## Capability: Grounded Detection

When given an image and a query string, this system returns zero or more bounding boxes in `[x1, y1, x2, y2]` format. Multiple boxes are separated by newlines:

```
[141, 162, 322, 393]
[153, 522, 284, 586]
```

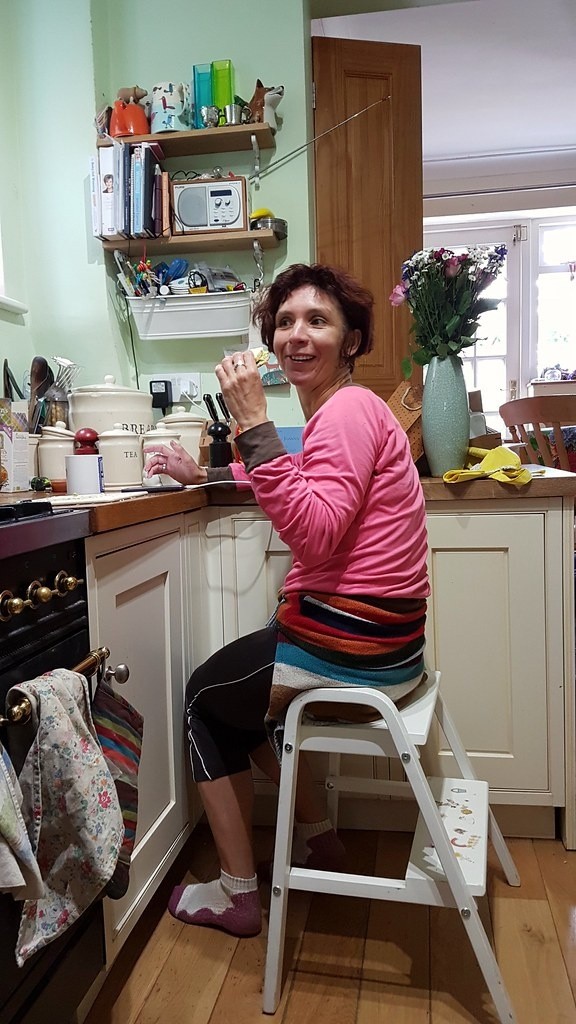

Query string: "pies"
[249, 347, 270, 367]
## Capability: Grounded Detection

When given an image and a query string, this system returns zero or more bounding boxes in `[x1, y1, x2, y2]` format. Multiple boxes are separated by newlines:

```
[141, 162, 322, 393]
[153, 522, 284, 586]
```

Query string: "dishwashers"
[0, 571, 130, 1011]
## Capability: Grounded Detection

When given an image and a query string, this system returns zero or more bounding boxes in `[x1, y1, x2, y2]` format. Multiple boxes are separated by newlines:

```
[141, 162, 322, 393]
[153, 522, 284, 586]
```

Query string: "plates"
[18, 491, 149, 505]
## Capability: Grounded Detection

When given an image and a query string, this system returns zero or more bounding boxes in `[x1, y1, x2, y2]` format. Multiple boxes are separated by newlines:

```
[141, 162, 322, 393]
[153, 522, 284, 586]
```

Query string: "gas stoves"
[0, 501, 93, 560]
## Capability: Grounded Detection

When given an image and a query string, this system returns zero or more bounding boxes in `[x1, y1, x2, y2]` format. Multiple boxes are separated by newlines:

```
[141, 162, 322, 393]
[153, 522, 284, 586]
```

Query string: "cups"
[27, 432, 42, 491]
[97, 405, 206, 489]
[108, 100, 149, 138]
[65, 454, 106, 494]
[37, 421, 77, 481]
[42, 400, 70, 429]
[192, 59, 252, 130]
[151, 80, 192, 134]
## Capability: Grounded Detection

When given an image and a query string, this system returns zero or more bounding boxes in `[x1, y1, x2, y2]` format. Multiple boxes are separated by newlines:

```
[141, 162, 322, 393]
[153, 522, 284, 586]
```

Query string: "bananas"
[249, 208, 275, 219]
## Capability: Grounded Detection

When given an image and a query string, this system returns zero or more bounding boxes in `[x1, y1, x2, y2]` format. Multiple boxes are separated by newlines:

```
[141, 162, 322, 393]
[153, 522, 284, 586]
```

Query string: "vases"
[423, 357, 470, 477]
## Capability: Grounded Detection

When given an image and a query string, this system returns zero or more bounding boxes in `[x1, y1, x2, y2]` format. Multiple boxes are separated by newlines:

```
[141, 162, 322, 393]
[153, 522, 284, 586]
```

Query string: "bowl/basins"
[69, 375, 155, 431]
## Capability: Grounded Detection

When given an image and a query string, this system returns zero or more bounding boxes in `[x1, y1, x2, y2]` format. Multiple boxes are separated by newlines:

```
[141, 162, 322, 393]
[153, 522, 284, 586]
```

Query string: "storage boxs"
[1, 431, 30, 493]
[130, 291, 252, 343]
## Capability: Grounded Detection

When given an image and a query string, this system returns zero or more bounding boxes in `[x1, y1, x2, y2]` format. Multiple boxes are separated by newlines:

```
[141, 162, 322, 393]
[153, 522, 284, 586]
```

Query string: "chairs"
[500, 392, 576, 471]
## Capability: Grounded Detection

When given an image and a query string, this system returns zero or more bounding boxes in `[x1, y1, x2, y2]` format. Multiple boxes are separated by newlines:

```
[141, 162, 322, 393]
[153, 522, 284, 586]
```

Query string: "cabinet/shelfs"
[85, 508, 213, 972]
[218, 499, 570, 842]
[97, 121, 279, 252]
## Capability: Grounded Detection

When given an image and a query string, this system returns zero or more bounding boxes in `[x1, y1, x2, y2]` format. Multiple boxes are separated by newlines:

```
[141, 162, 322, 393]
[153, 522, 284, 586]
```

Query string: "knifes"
[202, 392, 232, 431]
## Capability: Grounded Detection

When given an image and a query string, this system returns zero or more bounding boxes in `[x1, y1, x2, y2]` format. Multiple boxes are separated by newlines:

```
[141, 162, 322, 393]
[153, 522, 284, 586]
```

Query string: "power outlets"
[172, 372, 202, 404]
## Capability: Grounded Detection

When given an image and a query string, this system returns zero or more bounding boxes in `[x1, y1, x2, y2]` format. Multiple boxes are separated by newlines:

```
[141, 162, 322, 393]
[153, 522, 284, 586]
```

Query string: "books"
[92, 143, 171, 241]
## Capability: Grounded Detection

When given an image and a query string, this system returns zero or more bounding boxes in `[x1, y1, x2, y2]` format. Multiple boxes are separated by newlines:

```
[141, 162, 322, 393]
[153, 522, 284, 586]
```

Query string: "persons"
[136, 265, 432, 937]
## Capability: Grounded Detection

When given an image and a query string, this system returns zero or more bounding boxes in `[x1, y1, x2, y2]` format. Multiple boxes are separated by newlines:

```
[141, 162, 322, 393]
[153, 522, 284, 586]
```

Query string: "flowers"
[389, 245, 507, 379]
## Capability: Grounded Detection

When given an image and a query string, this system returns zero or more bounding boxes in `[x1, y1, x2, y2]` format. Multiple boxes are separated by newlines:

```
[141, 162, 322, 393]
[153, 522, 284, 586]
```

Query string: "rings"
[162, 463, 166, 473]
[233, 362, 247, 372]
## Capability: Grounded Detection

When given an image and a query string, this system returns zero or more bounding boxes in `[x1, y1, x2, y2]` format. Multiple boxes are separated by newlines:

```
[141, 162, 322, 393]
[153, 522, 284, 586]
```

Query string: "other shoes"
[266, 828, 347, 882]
[165, 884, 261, 937]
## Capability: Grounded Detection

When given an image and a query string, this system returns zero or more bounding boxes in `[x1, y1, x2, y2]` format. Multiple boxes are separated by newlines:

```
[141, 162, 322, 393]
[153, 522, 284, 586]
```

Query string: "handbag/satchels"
[386, 380, 426, 462]
